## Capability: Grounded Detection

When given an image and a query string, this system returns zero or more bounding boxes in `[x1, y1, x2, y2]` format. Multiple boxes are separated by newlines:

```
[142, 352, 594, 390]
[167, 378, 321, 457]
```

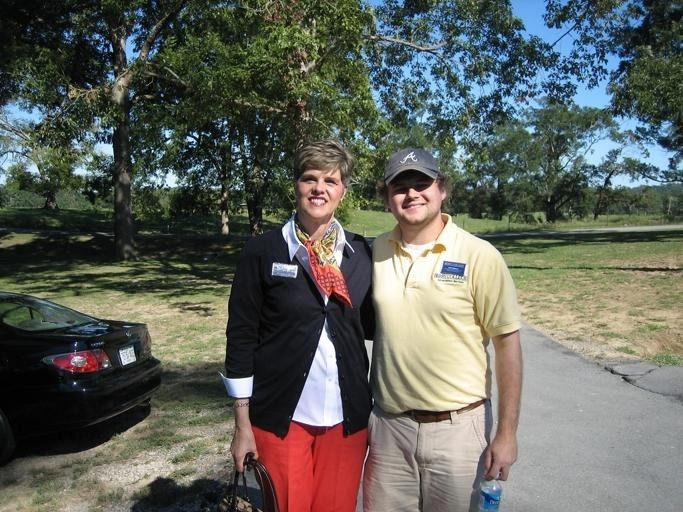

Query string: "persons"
[363, 148, 523, 512]
[218, 140, 375, 512]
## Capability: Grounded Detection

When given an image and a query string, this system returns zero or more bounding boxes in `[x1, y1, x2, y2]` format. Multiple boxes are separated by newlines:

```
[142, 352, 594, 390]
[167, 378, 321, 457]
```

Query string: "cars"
[0, 287, 166, 468]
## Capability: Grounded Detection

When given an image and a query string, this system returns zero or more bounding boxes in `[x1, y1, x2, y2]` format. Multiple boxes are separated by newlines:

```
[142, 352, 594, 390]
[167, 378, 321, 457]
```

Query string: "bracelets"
[231, 401, 249, 409]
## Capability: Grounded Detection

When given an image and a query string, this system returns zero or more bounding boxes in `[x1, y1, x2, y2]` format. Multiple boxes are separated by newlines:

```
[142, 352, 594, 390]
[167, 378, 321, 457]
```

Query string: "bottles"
[476, 476, 502, 512]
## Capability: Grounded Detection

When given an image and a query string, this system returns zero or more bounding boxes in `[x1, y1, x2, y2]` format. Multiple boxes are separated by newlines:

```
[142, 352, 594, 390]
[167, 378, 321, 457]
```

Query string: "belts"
[408, 400, 489, 421]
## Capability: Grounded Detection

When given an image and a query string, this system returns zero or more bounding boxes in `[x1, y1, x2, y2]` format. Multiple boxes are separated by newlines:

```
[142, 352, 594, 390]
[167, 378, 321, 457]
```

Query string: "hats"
[381, 148, 437, 184]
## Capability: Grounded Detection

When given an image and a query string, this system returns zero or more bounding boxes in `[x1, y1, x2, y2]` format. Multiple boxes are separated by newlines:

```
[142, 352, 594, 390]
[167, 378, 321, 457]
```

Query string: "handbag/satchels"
[219, 493, 261, 512]
